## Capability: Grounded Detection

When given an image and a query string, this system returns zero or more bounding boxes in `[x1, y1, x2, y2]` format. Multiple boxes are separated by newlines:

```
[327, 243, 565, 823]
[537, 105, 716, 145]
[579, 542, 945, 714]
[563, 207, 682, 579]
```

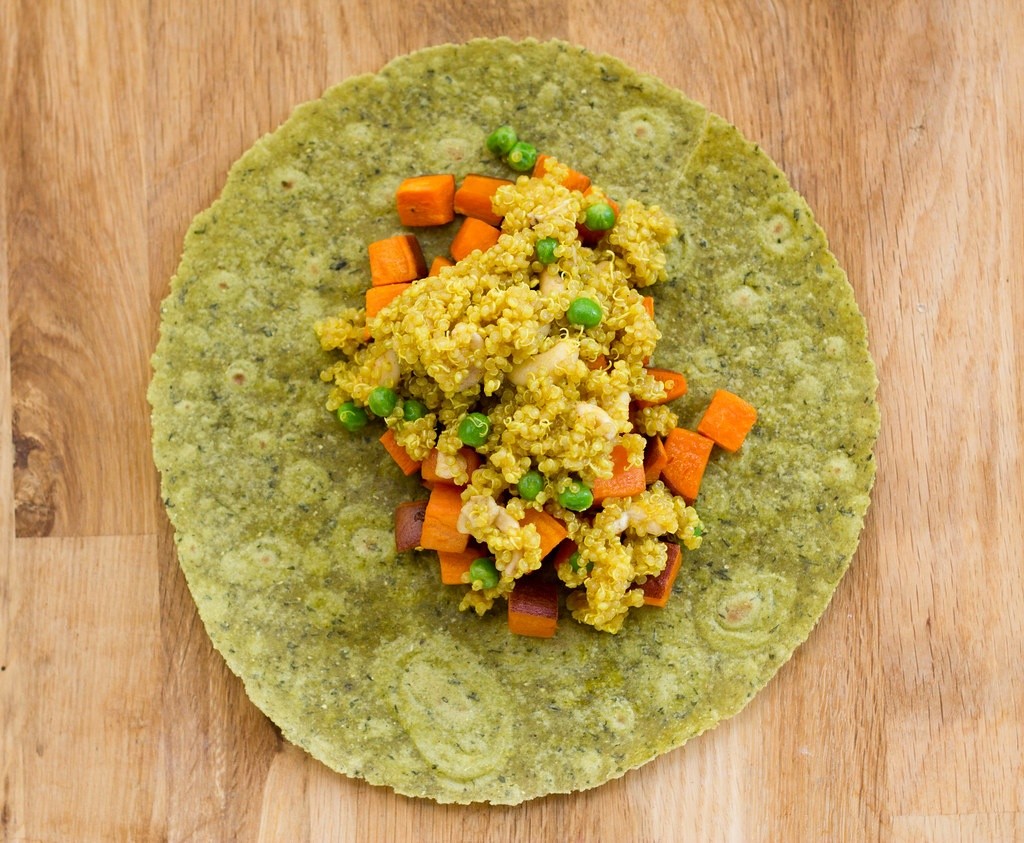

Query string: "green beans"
[336, 126, 615, 590]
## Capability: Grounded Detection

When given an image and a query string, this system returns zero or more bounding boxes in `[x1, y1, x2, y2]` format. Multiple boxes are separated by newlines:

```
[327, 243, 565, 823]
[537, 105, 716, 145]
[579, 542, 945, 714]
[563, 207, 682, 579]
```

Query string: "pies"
[146, 33, 883, 809]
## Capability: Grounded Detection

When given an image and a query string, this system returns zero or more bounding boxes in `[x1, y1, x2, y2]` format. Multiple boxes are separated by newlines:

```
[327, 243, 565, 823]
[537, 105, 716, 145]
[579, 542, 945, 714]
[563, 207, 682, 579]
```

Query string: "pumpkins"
[365, 157, 757, 640]
